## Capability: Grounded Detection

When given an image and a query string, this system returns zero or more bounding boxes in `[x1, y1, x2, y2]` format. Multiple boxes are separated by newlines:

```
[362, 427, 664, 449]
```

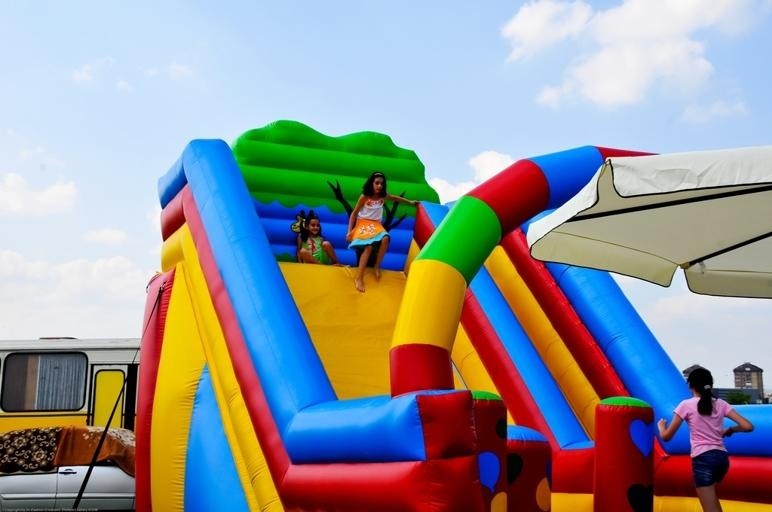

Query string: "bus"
[0, 334, 140, 435]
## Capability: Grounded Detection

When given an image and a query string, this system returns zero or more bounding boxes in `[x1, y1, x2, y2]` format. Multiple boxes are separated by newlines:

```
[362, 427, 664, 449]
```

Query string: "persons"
[346, 172, 421, 292]
[657, 369, 753, 512]
[297, 216, 343, 266]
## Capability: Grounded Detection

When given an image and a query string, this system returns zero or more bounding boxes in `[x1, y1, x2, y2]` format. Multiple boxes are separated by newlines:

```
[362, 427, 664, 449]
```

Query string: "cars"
[0, 426, 138, 512]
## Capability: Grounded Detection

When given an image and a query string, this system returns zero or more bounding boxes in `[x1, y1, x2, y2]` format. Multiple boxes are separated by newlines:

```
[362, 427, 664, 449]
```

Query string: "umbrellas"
[526, 146, 772, 299]
